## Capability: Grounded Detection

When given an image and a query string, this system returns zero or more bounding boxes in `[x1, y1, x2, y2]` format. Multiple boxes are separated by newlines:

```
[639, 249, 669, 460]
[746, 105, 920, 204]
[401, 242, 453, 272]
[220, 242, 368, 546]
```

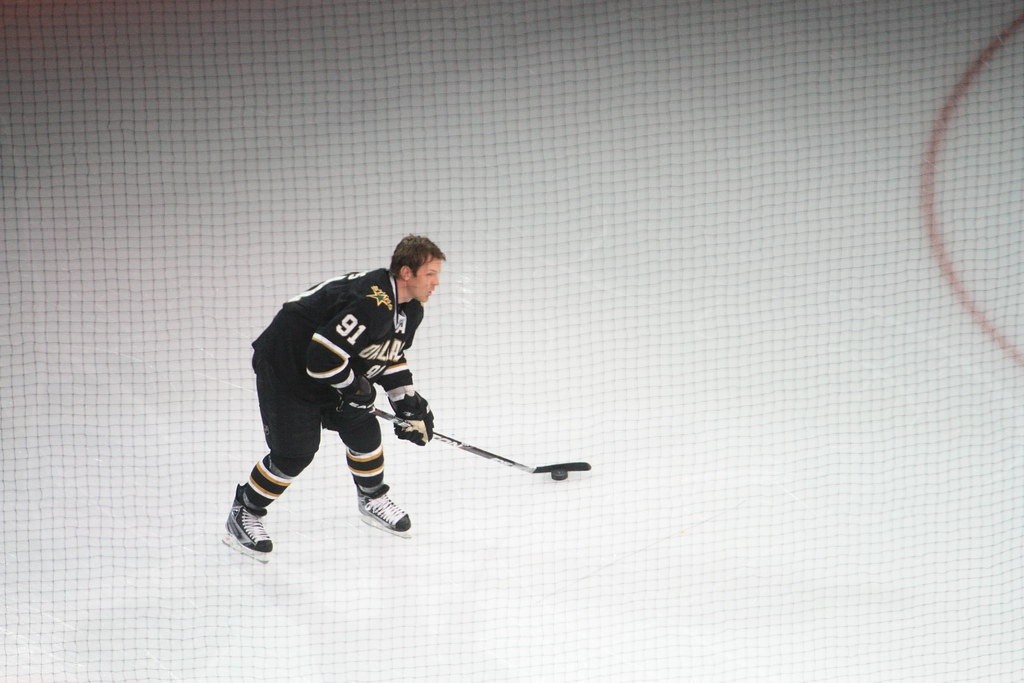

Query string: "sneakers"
[357, 486, 411, 538]
[222, 483, 273, 562]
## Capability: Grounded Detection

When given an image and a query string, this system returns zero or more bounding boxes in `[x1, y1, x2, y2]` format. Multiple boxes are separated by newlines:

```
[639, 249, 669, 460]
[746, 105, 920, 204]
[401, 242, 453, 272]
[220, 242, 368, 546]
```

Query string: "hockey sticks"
[370, 405, 593, 476]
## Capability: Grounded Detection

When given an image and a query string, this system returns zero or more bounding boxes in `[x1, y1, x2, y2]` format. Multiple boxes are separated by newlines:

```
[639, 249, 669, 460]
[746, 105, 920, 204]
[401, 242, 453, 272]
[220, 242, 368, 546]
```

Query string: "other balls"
[550, 468, 569, 482]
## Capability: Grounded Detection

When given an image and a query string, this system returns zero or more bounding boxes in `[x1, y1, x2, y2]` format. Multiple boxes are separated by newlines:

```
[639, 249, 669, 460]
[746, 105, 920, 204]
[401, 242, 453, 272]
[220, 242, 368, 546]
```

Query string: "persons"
[222, 235, 446, 562]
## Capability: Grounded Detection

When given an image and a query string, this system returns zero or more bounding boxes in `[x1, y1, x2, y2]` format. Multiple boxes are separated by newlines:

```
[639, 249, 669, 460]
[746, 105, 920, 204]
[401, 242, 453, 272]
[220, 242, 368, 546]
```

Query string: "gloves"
[388, 391, 434, 446]
[327, 372, 376, 413]
